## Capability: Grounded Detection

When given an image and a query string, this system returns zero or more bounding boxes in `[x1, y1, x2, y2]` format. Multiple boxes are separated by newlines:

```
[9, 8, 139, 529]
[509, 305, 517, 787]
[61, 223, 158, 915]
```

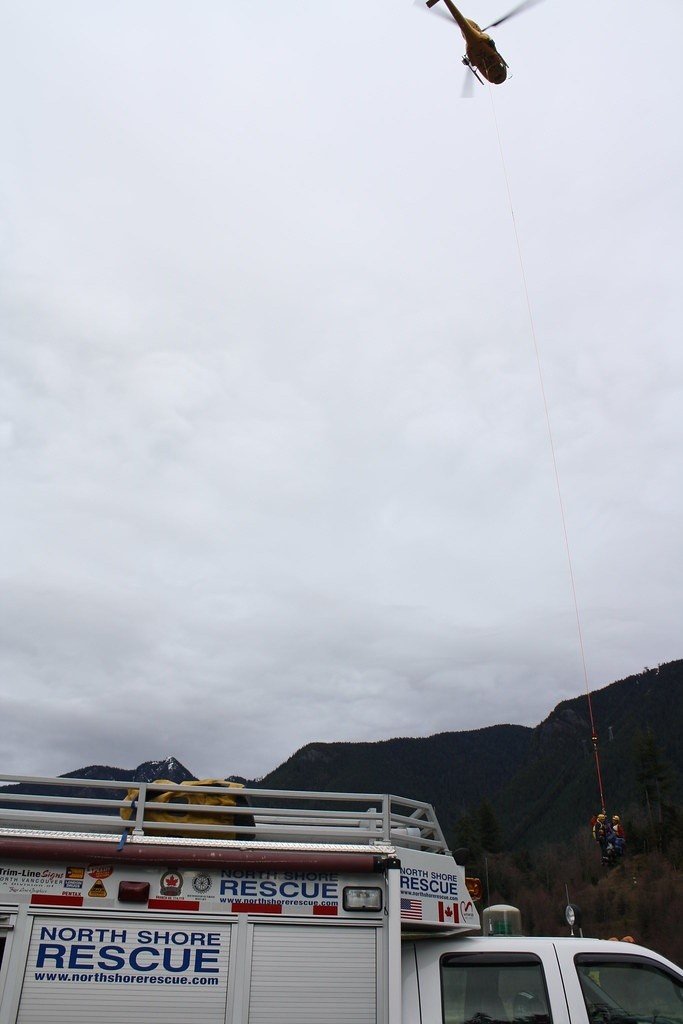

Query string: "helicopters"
[425, 0, 540, 88]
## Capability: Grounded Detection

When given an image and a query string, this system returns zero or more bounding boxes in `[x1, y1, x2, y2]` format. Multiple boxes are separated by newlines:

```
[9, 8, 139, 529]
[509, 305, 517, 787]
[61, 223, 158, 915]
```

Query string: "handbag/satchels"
[119, 779, 258, 842]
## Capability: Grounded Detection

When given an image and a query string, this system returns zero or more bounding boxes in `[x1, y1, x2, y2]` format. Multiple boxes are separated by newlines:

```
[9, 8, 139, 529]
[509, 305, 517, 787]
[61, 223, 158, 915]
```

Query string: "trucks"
[0, 771, 683, 1023]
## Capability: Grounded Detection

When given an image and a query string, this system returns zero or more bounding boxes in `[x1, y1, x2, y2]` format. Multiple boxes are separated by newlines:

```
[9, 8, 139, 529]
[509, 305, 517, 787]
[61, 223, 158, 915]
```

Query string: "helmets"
[612, 816, 619, 820]
[609, 937, 618, 941]
[598, 814, 605, 819]
[622, 936, 635, 943]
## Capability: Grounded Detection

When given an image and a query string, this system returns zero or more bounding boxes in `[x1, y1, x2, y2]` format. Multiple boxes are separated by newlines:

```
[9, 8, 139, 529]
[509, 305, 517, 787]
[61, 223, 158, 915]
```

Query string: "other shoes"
[600, 858, 604, 864]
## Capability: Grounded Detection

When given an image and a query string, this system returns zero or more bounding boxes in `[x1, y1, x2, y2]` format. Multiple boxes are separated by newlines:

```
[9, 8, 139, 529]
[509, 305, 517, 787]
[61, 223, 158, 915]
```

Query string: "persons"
[592, 811, 625, 867]
